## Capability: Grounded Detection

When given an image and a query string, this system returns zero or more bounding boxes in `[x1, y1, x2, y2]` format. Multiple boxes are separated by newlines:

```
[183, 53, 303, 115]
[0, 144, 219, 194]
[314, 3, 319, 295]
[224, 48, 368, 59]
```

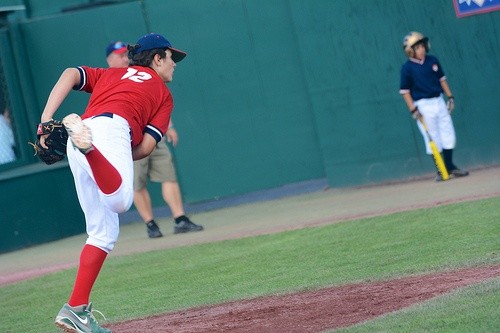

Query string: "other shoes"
[437, 170, 441, 181]
[451, 167, 469, 177]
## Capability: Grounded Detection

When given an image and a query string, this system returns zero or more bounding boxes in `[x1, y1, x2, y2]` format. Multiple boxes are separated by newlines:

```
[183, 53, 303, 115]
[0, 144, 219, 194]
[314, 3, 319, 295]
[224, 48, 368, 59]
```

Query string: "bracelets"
[447, 96, 454, 99]
[168, 125, 176, 129]
[408, 105, 418, 114]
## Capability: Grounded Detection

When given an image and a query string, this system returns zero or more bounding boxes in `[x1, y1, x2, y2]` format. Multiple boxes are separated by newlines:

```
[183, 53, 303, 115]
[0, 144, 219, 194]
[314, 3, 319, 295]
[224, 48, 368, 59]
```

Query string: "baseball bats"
[419, 115, 449, 181]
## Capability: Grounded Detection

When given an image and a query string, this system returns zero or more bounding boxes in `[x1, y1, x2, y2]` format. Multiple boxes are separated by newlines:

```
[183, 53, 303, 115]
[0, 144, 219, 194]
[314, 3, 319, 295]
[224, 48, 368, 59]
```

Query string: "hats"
[106, 41, 129, 57]
[128, 32, 187, 64]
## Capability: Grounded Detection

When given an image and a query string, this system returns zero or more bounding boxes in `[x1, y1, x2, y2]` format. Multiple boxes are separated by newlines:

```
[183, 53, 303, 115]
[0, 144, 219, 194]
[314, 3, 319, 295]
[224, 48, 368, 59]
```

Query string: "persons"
[33, 33, 187, 333]
[399, 30, 469, 182]
[104, 41, 203, 239]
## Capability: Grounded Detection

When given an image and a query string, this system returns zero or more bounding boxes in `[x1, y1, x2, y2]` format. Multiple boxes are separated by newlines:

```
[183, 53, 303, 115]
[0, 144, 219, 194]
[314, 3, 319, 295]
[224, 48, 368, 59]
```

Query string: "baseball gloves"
[29, 120, 69, 164]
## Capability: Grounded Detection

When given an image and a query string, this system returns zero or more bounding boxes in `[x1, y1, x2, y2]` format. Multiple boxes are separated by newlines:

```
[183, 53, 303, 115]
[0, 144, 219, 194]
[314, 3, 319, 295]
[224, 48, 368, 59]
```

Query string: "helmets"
[402, 31, 431, 58]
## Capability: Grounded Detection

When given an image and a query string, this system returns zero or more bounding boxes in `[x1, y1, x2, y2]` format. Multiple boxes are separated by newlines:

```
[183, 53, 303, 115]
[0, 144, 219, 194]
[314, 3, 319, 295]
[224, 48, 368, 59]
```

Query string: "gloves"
[412, 108, 423, 121]
[447, 97, 455, 114]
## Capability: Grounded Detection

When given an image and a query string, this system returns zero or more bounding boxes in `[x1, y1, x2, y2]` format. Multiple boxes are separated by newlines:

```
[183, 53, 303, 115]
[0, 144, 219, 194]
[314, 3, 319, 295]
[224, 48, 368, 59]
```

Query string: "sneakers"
[147, 225, 163, 238]
[53, 303, 113, 333]
[62, 113, 94, 152]
[174, 220, 204, 234]
